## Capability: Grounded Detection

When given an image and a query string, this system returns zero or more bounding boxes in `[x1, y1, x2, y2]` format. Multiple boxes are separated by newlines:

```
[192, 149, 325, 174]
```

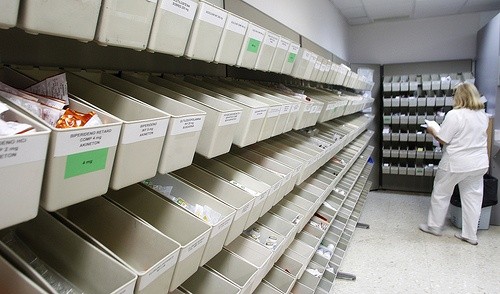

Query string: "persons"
[418, 81, 490, 245]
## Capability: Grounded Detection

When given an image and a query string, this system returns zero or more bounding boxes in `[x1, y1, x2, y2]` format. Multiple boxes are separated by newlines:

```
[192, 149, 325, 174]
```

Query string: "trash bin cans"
[449, 173, 499, 230]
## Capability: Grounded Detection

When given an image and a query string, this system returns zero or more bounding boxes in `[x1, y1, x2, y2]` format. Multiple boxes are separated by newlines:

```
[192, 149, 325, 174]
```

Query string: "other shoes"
[454, 231, 479, 245]
[419, 224, 441, 236]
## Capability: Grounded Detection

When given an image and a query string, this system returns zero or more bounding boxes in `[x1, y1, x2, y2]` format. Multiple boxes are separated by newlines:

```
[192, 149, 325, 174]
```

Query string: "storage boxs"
[0, 0, 376, 294]
[381, 72, 475, 178]
